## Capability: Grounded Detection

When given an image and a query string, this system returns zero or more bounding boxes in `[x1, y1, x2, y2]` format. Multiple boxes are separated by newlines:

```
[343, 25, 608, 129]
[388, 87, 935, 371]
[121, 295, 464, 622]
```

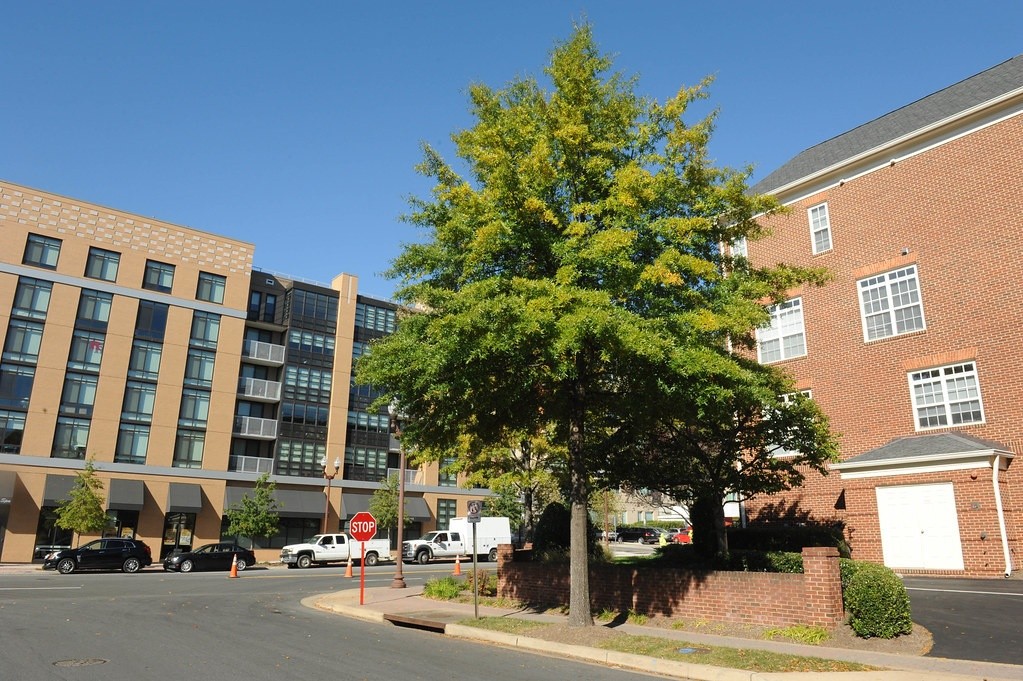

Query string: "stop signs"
[350, 512, 376, 542]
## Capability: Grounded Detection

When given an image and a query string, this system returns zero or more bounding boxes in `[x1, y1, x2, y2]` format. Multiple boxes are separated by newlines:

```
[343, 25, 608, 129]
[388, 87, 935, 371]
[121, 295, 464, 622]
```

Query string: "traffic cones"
[344, 556, 354, 579]
[451, 554, 464, 575]
[227, 554, 241, 578]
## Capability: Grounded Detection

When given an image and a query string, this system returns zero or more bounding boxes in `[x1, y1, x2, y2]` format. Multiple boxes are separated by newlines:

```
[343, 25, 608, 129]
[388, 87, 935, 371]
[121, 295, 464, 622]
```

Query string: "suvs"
[42, 537, 152, 574]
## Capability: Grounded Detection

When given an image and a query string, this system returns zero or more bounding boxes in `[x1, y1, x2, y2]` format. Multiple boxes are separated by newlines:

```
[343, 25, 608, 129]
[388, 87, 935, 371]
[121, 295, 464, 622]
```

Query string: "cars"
[162, 539, 255, 573]
[594, 529, 618, 540]
[616, 527, 692, 544]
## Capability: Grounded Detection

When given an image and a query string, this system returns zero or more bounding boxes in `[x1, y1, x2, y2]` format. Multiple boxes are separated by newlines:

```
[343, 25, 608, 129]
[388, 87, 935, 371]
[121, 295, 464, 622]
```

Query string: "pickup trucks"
[279, 534, 390, 569]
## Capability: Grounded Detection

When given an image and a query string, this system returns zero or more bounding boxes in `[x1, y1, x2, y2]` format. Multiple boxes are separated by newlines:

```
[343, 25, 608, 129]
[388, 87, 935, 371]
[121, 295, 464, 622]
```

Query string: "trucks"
[401, 516, 512, 565]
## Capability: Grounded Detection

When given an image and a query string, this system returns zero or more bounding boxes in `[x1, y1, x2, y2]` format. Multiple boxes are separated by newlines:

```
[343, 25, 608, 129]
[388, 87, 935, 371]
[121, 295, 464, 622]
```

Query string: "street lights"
[388, 397, 419, 588]
[319, 456, 341, 567]
